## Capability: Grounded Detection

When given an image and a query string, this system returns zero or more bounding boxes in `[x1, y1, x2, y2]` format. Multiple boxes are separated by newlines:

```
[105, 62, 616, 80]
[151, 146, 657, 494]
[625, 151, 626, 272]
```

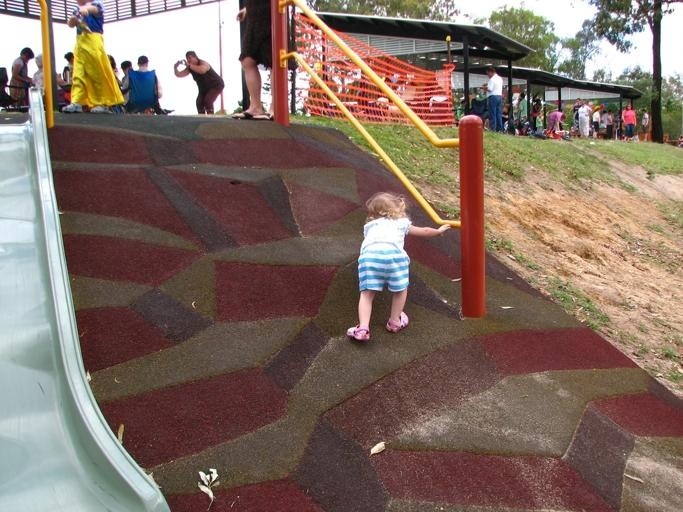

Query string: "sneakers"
[91, 105, 108, 113]
[62, 104, 82, 112]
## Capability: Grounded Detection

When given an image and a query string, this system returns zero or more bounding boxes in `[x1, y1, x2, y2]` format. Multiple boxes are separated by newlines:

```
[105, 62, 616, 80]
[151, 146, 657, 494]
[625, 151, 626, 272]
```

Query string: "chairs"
[0, 67, 25, 112]
[122, 69, 158, 114]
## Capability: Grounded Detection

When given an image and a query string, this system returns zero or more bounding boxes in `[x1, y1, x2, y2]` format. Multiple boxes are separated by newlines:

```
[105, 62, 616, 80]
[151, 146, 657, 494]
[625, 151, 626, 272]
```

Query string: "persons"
[62, 0, 124, 113]
[347, 192, 451, 341]
[10, 47, 162, 106]
[174, 51, 225, 115]
[458, 67, 652, 143]
[232, 0, 299, 121]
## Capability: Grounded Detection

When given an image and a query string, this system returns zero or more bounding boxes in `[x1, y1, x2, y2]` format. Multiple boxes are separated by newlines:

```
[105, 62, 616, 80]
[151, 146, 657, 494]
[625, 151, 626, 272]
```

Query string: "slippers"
[232, 111, 269, 119]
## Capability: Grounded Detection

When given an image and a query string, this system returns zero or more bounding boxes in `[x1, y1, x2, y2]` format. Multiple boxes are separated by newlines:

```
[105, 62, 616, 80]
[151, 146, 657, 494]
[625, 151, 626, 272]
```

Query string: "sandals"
[346, 325, 370, 341]
[387, 312, 409, 332]
